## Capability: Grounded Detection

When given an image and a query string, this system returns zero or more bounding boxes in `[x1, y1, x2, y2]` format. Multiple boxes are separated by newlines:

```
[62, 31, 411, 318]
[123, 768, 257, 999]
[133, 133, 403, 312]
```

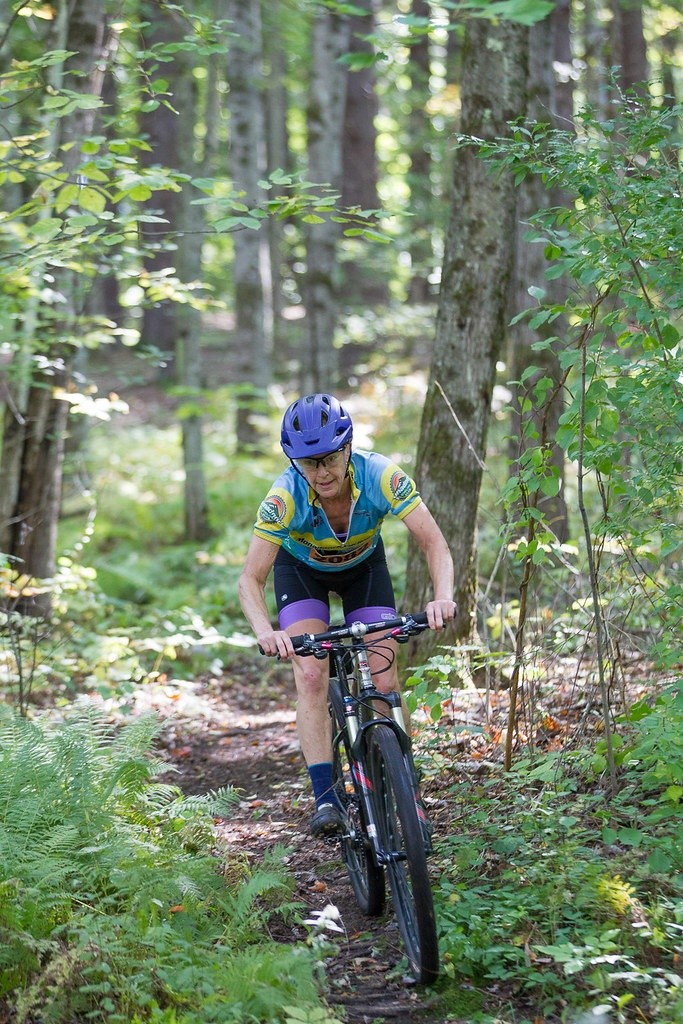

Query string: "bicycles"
[260, 606, 458, 988]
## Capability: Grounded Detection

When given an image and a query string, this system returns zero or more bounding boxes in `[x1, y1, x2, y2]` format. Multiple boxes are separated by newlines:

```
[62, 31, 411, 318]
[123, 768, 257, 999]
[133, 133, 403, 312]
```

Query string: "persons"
[237, 393, 456, 857]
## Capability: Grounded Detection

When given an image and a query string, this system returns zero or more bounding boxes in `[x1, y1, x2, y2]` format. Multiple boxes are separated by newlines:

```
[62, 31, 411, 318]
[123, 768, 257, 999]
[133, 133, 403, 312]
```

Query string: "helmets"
[280, 393, 353, 459]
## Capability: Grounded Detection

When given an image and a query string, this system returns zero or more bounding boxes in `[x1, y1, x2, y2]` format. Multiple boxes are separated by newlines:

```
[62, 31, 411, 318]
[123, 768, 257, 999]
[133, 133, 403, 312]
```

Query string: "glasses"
[297, 448, 344, 470]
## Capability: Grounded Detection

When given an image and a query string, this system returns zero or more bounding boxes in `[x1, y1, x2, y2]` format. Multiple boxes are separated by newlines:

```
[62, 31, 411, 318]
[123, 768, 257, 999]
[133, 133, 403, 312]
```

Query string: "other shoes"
[311, 807, 349, 842]
[420, 797, 433, 836]
